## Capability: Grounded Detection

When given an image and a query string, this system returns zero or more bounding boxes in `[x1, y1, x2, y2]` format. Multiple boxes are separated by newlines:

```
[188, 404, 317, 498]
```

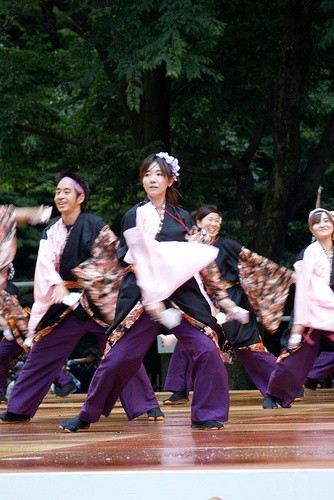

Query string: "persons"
[0, 203, 101, 403]
[58, 152, 230, 431]
[162, 206, 297, 405]
[262, 185, 334, 409]
[0, 172, 165, 425]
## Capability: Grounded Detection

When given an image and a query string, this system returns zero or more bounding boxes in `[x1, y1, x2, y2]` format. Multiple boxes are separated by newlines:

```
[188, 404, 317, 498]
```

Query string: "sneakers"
[163, 391, 190, 405]
[303, 378, 318, 393]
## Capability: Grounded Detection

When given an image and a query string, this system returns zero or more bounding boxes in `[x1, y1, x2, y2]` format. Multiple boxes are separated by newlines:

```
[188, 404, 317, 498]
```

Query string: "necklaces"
[322, 246, 333, 258]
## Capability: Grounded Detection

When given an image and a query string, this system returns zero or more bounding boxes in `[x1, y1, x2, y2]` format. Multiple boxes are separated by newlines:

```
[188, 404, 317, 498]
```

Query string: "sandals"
[293, 387, 306, 401]
[262, 397, 278, 410]
[190, 420, 225, 430]
[0, 412, 32, 422]
[55, 380, 77, 399]
[0, 396, 8, 404]
[58, 417, 90, 433]
[147, 407, 166, 421]
